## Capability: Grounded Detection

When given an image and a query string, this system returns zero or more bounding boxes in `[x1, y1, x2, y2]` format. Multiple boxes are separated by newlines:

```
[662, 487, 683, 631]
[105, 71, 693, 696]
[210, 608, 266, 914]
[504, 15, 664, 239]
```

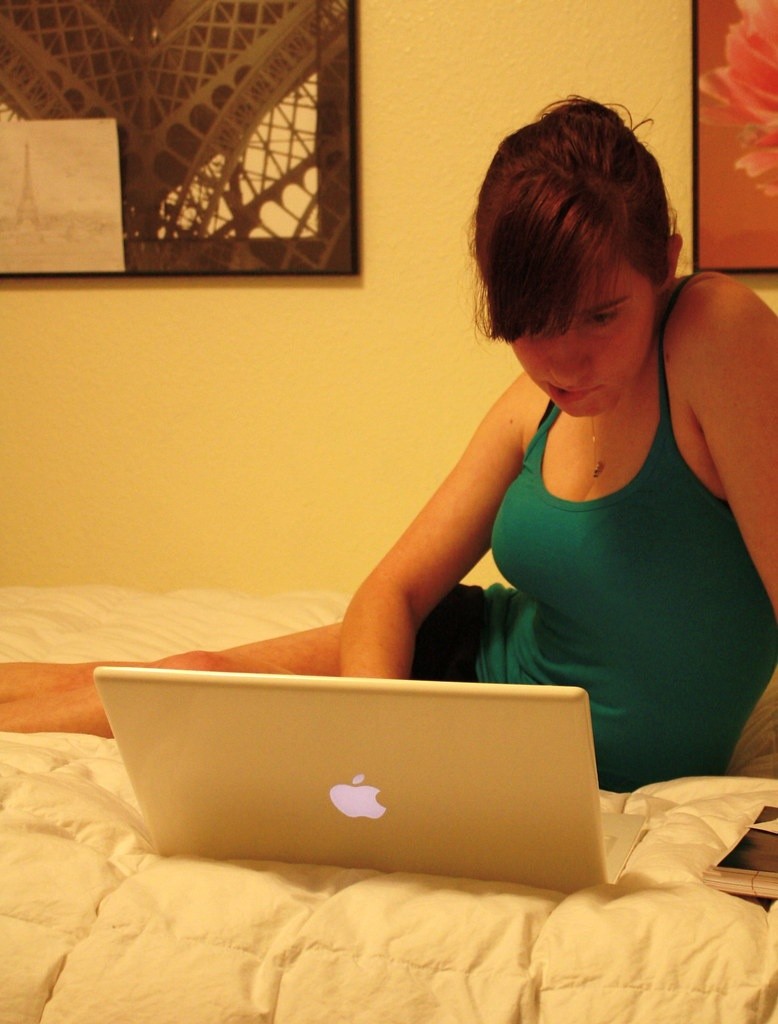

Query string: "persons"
[0, 95, 778, 793]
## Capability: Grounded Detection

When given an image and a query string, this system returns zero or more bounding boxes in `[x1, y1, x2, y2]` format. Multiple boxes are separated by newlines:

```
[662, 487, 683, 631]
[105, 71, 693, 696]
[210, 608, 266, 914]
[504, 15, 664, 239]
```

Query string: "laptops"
[94, 665, 648, 895]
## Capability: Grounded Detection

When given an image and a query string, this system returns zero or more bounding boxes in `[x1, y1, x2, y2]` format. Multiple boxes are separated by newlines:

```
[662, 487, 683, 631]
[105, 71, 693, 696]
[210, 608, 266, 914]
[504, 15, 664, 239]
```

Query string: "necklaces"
[592, 404, 653, 477]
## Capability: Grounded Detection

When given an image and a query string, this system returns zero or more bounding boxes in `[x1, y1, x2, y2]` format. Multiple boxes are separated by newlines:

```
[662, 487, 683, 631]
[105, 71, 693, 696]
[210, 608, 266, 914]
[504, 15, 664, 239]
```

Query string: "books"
[701, 805, 778, 900]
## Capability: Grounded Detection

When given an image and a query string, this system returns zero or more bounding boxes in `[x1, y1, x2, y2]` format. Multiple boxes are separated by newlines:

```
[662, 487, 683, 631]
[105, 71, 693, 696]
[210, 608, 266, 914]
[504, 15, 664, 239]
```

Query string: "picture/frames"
[692, 0, 778, 276]
[0, 0, 362, 278]
